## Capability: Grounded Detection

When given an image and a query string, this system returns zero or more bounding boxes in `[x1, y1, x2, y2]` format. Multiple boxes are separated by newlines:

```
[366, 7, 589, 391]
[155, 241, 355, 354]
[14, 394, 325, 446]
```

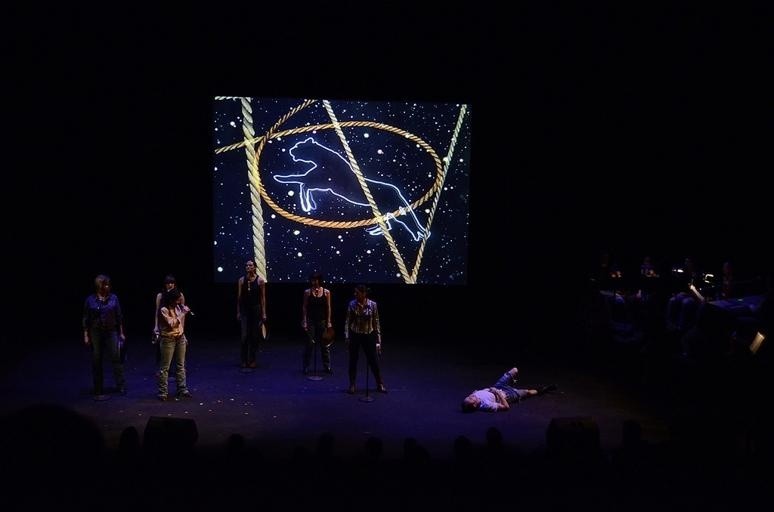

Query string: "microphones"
[179, 304, 195, 317]
[363, 302, 373, 313]
[312, 283, 319, 292]
[97, 296, 104, 308]
[245, 271, 251, 279]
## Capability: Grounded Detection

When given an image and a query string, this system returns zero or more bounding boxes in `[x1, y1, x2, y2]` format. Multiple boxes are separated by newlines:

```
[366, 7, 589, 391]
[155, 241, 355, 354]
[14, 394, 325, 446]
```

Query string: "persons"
[235, 260, 268, 368]
[154, 289, 192, 401]
[598, 256, 734, 313]
[462, 365, 557, 414]
[342, 284, 388, 394]
[80, 273, 129, 400]
[151, 276, 188, 376]
[299, 272, 334, 374]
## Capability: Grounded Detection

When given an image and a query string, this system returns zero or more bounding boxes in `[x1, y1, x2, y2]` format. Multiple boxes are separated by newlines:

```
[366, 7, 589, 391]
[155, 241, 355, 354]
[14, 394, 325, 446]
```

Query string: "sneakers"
[239, 359, 248, 368]
[156, 391, 169, 401]
[346, 382, 357, 394]
[248, 358, 257, 368]
[375, 384, 388, 394]
[177, 389, 193, 399]
